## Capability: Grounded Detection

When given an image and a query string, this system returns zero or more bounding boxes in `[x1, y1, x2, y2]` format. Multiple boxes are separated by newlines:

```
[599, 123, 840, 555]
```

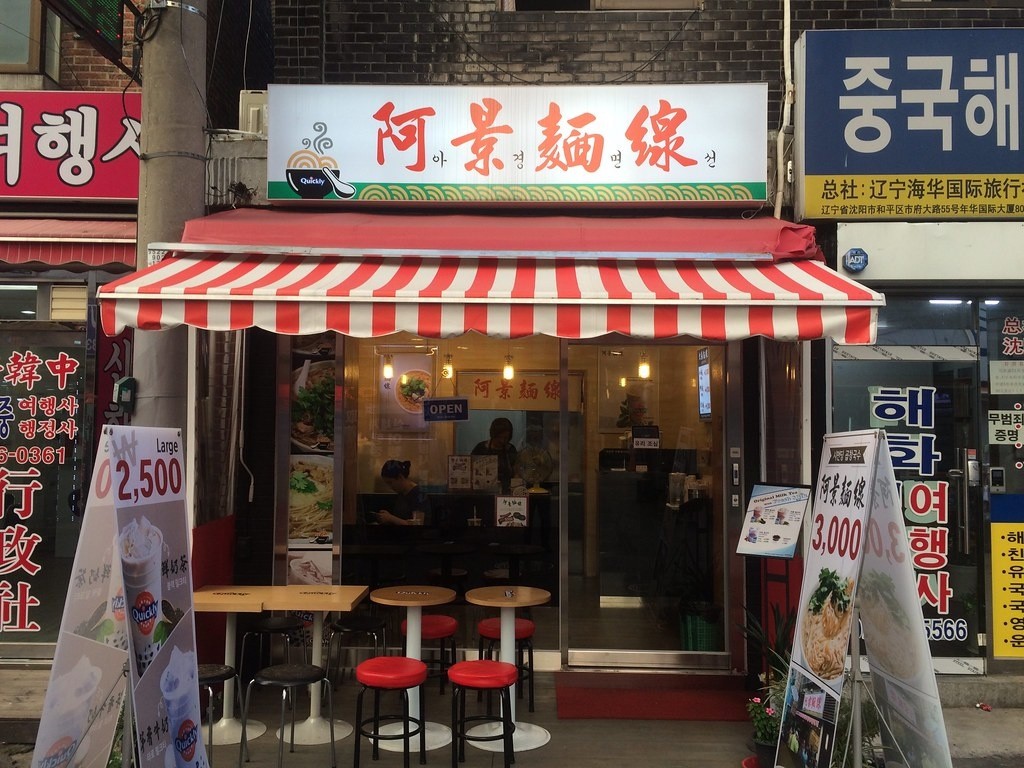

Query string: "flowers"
[746, 697, 786, 744]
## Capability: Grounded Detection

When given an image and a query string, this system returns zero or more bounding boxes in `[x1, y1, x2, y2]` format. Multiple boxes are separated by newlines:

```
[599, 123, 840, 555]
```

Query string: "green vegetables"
[402, 377, 425, 395]
[808, 567, 853, 610]
[290, 471, 333, 509]
[289, 373, 337, 439]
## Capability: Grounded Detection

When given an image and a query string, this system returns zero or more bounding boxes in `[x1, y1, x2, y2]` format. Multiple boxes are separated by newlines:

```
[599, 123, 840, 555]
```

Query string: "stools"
[321, 615, 389, 709]
[447, 660, 519, 768]
[235, 616, 310, 711]
[353, 657, 428, 768]
[399, 614, 459, 700]
[196, 664, 250, 768]
[476, 618, 536, 718]
[238, 663, 337, 768]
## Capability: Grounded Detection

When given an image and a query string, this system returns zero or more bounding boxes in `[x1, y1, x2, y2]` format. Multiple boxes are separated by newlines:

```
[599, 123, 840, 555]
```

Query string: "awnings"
[95, 244, 884, 345]
[0, 219, 137, 267]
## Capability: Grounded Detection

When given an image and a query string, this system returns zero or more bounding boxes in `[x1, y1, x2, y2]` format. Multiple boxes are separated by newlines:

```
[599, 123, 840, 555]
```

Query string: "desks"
[464, 586, 551, 753]
[341, 541, 548, 590]
[262, 586, 370, 745]
[192, 584, 287, 746]
[369, 585, 456, 752]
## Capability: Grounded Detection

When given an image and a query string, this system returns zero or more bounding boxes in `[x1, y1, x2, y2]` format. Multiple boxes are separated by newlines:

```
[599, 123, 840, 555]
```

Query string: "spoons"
[322, 167, 356, 199]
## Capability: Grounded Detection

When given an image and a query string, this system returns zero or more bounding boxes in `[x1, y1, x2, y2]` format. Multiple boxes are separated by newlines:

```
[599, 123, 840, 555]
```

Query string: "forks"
[285, 169, 340, 199]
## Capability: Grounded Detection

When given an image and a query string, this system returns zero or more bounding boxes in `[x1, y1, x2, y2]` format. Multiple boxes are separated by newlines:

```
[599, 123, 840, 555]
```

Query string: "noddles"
[289, 461, 335, 537]
[802, 577, 852, 679]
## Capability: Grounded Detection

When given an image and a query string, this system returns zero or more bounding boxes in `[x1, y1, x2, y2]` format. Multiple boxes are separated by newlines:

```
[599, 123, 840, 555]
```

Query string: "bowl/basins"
[288, 455, 333, 544]
[396, 369, 432, 414]
[290, 360, 334, 453]
[800, 579, 843, 687]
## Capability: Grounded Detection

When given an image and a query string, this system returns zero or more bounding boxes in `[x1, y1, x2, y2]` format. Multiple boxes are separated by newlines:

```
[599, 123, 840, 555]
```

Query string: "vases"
[748, 730, 779, 768]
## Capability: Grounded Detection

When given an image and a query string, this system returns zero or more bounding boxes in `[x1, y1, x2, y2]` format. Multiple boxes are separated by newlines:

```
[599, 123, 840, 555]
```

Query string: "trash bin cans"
[679, 597, 723, 651]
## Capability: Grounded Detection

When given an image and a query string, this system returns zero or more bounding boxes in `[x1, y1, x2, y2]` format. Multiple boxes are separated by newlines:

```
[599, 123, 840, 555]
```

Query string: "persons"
[470, 418, 518, 493]
[375, 460, 430, 580]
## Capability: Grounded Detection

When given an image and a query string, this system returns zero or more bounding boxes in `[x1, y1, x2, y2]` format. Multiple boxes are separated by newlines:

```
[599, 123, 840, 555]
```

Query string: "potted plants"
[734, 601, 799, 713]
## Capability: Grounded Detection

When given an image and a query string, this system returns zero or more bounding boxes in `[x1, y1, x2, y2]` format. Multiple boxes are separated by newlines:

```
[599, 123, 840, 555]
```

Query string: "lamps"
[502, 340, 515, 381]
[638, 338, 651, 379]
[382, 335, 395, 379]
[441, 339, 454, 379]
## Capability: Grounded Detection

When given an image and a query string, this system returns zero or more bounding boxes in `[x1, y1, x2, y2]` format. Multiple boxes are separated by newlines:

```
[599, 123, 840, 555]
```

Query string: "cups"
[669, 473, 709, 505]
[407, 520, 417, 525]
[104, 536, 128, 650]
[32, 665, 102, 768]
[777, 510, 788, 525]
[120, 524, 163, 677]
[467, 518, 482, 527]
[287, 560, 327, 625]
[161, 652, 203, 768]
[748, 528, 760, 543]
[754, 509, 764, 522]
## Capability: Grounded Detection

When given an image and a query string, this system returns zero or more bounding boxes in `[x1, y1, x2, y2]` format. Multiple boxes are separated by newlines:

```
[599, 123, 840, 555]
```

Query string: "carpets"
[554, 671, 770, 723]
